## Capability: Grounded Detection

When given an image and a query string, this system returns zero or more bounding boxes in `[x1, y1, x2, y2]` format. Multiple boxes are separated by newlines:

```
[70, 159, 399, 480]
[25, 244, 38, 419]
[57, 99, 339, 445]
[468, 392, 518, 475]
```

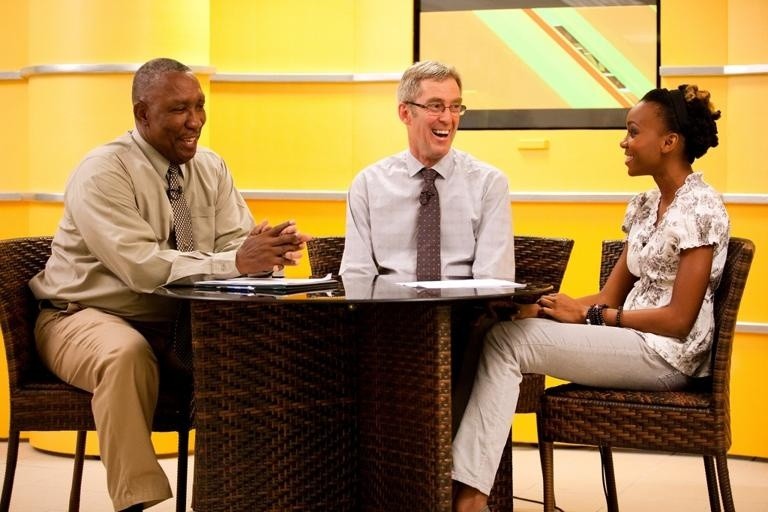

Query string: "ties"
[169, 164, 194, 367]
[417, 169, 441, 281]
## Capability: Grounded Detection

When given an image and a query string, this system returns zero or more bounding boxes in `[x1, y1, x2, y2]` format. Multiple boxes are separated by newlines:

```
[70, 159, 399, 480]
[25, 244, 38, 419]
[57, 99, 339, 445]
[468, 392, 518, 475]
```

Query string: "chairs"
[306, 236, 345, 298]
[481, 236, 582, 512]
[0, 237, 190, 512]
[539, 237, 755, 512]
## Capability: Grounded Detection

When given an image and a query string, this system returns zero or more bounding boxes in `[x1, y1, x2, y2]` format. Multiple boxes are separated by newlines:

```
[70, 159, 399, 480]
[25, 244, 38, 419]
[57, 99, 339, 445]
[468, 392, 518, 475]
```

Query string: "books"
[193, 275, 339, 295]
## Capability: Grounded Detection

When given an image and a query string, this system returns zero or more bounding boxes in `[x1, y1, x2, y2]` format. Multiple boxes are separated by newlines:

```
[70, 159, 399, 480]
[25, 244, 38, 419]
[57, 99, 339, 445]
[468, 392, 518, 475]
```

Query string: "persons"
[338, 56, 516, 284]
[451, 84, 731, 512]
[28, 59, 312, 511]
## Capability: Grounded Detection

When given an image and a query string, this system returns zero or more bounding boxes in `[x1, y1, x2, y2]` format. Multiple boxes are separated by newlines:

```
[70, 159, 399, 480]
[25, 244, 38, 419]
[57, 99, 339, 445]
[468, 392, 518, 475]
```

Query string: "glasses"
[407, 102, 466, 117]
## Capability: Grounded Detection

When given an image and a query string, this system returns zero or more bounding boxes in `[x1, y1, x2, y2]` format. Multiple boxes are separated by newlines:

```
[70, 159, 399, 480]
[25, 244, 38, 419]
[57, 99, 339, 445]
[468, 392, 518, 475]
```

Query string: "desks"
[154, 274, 554, 512]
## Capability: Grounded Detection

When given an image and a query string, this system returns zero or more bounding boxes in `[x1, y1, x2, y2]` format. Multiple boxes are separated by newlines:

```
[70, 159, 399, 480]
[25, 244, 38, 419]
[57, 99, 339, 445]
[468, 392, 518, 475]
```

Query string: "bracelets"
[615, 306, 624, 326]
[586, 303, 609, 326]
